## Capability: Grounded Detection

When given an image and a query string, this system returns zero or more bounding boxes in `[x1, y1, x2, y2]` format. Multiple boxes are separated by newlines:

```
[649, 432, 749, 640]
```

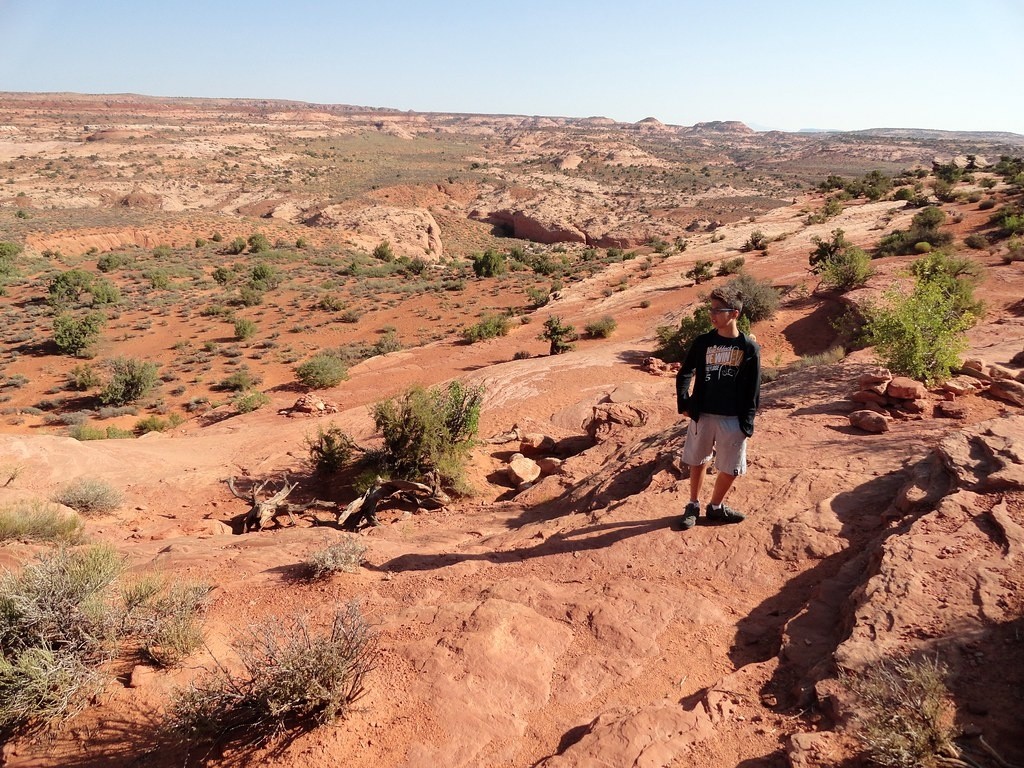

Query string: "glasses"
[707, 307, 735, 315]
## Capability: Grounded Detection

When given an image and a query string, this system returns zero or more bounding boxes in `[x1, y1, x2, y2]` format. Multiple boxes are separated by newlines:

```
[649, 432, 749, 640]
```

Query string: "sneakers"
[705, 503, 745, 523]
[681, 499, 700, 528]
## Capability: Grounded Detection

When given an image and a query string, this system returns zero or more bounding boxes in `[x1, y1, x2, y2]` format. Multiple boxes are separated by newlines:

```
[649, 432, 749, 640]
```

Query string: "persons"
[675, 285, 760, 528]
